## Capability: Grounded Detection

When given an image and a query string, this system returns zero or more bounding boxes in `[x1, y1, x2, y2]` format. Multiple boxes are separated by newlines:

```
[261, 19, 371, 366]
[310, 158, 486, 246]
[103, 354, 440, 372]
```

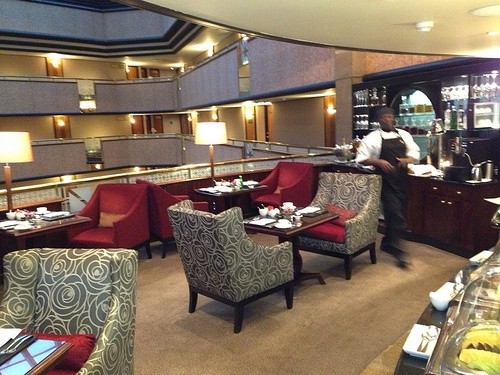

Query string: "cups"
[6, 207, 47, 221]
[259, 202, 301, 222]
[400, 104, 433, 113]
[215, 179, 240, 191]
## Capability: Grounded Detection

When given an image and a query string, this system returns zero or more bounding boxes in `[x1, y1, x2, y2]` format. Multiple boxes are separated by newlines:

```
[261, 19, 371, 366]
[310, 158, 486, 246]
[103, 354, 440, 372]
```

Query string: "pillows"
[21, 331, 95, 371]
[328, 205, 357, 227]
[98, 211, 126, 227]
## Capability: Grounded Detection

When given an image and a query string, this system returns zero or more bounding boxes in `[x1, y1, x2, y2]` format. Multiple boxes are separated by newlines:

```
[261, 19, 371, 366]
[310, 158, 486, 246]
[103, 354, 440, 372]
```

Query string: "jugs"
[471, 163, 483, 181]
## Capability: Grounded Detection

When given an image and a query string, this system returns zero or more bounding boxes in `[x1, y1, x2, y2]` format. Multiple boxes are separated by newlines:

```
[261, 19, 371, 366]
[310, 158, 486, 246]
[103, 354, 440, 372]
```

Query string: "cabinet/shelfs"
[351, 58, 500, 168]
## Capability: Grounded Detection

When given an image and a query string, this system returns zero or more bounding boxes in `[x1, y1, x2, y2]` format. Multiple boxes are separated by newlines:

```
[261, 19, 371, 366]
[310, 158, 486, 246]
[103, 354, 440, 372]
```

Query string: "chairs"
[0, 247, 138, 375]
[249, 161, 313, 208]
[296, 172, 383, 280]
[135, 179, 208, 258]
[168, 199, 295, 334]
[59, 183, 152, 260]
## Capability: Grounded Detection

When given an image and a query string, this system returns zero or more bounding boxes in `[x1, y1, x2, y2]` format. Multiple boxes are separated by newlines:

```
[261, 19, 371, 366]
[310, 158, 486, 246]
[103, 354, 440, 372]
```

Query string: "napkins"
[0, 334, 38, 366]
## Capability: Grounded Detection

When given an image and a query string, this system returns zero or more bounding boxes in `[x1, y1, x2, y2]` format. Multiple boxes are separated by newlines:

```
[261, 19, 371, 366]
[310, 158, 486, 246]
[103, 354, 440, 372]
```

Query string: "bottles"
[239, 176, 243, 188]
[444, 102, 467, 130]
[485, 160, 493, 180]
[354, 87, 386, 107]
[394, 118, 434, 135]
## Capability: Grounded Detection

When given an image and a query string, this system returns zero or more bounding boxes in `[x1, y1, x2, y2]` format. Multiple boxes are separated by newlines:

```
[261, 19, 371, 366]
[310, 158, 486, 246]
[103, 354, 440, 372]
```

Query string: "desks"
[0, 215, 92, 250]
[0, 339, 74, 375]
[194, 185, 268, 214]
[243, 211, 339, 285]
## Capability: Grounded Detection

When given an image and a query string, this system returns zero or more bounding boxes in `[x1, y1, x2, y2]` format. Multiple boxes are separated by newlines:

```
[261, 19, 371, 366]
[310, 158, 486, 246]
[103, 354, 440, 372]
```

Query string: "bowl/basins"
[335, 149, 353, 161]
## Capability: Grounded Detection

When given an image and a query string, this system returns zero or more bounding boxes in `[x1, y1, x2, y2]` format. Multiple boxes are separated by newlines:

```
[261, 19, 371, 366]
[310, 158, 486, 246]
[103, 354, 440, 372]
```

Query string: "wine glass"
[354, 114, 368, 129]
[441, 85, 469, 100]
[471, 73, 500, 98]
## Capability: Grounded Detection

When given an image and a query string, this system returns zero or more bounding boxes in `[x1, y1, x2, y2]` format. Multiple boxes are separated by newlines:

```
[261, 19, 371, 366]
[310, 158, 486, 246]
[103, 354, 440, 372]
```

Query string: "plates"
[402, 324, 441, 359]
[249, 206, 321, 229]
[198, 181, 258, 193]
[469, 251, 494, 265]
[0, 212, 70, 230]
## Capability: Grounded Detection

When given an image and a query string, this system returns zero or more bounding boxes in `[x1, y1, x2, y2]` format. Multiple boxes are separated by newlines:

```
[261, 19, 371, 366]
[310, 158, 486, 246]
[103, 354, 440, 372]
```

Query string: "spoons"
[0, 335, 33, 354]
[416, 326, 439, 353]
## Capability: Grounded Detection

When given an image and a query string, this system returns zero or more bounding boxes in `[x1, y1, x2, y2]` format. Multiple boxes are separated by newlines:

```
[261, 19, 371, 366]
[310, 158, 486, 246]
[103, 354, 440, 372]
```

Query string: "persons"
[355, 106, 421, 268]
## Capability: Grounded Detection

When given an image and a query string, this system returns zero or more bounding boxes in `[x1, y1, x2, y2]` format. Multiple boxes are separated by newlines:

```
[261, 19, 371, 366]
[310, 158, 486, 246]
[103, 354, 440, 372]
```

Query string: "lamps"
[0, 131, 33, 211]
[194, 122, 228, 186]
